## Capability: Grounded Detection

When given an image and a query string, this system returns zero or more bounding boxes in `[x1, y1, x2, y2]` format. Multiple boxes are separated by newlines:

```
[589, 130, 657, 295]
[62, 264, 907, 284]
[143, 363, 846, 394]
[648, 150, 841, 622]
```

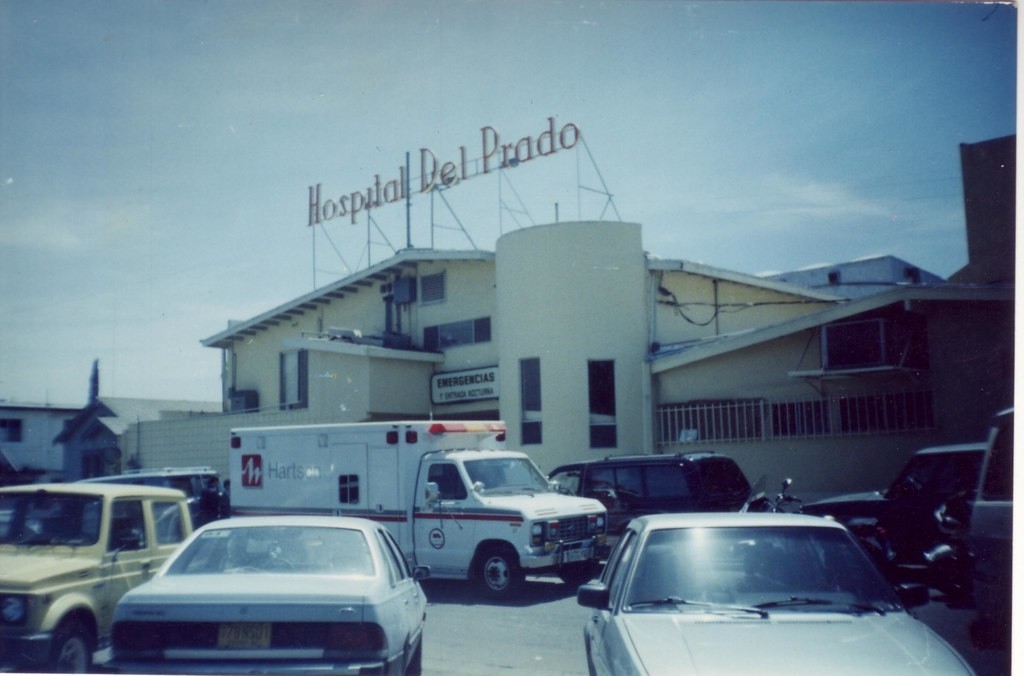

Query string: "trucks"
[226, 420, 608, 600]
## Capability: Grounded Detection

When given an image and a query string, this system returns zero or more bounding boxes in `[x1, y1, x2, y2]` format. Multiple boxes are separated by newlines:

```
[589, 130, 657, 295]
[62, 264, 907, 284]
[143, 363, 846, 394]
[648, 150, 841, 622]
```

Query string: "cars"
[1, 482, 428, 673]
[578, 407, 1011, 676]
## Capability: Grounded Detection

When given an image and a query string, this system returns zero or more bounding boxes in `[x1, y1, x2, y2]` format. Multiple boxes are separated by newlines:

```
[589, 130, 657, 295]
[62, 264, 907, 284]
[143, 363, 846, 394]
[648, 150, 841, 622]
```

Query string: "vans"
[549, 450, 752, 560]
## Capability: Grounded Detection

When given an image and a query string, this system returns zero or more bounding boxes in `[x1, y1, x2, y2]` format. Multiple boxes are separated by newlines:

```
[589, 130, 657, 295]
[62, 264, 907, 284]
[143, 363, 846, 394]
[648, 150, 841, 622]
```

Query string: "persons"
[199, 477, 222, 527]
[218, 480, 230, 519]
